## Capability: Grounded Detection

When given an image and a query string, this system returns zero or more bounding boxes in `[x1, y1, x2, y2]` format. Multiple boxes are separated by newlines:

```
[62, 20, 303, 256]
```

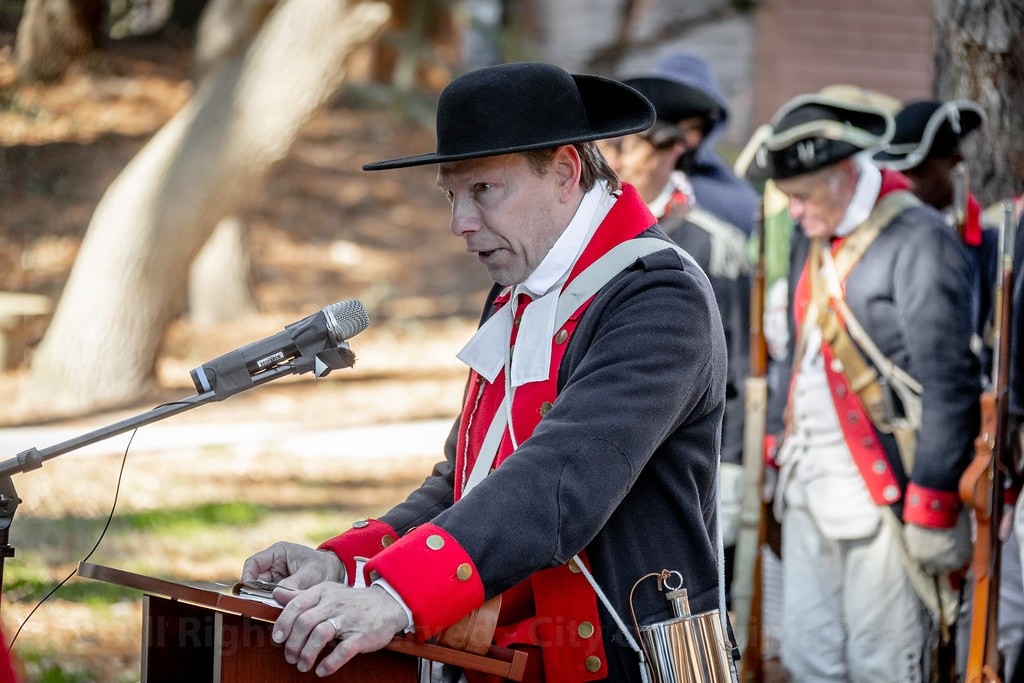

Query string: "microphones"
[188, 298, 370, 394]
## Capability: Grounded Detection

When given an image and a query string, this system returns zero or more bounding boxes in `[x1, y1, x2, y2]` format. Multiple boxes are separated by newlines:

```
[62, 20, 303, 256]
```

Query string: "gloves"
[902, 524, 975, 576]
[716, 463, 745, 551]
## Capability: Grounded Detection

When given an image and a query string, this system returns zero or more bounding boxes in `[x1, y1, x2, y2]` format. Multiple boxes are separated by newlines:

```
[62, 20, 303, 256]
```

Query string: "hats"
[731, 89, 896, 180]
[618, 74, 730, 153]
[871, 93, 991, 171]
[360, 61, 658, 171]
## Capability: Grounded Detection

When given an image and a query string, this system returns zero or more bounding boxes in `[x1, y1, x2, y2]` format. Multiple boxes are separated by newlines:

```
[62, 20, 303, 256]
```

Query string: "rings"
[324, 619, 343, 638]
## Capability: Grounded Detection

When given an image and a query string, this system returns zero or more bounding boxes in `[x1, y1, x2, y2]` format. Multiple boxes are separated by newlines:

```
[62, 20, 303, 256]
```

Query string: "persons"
[239, 62, 742, 683]
[585, 53, 1024, 683]
